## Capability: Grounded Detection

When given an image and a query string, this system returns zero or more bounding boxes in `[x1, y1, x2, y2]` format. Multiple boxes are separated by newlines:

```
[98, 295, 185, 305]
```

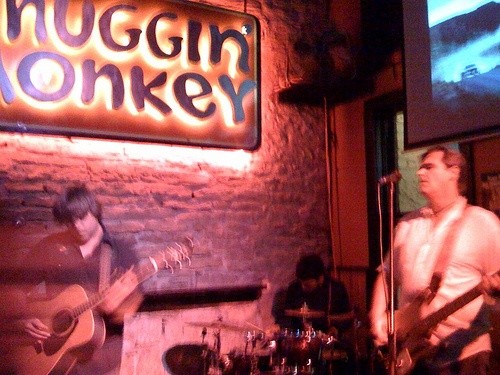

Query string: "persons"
[0, 178, 145, 375]
[282, 254, 357, 375]
[370, 144, 500, 375]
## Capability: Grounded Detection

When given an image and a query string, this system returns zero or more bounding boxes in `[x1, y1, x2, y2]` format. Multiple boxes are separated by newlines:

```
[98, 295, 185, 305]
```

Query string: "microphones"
[376, 169, 402, 187]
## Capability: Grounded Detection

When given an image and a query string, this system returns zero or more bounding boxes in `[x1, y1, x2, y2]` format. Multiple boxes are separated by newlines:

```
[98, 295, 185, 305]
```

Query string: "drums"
[223, 330, 352, 375]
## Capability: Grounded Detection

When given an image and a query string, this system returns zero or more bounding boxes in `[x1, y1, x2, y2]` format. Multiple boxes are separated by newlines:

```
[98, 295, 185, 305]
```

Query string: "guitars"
[8, 236, 194, 375]
[371, 271, 500, 375]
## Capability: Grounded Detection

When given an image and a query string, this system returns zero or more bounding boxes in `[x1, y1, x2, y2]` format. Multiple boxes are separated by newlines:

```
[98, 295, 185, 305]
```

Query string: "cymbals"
[163, 344, 215, 375]
[286, 307, 323, 318]
[188, 322, 244, 332]
